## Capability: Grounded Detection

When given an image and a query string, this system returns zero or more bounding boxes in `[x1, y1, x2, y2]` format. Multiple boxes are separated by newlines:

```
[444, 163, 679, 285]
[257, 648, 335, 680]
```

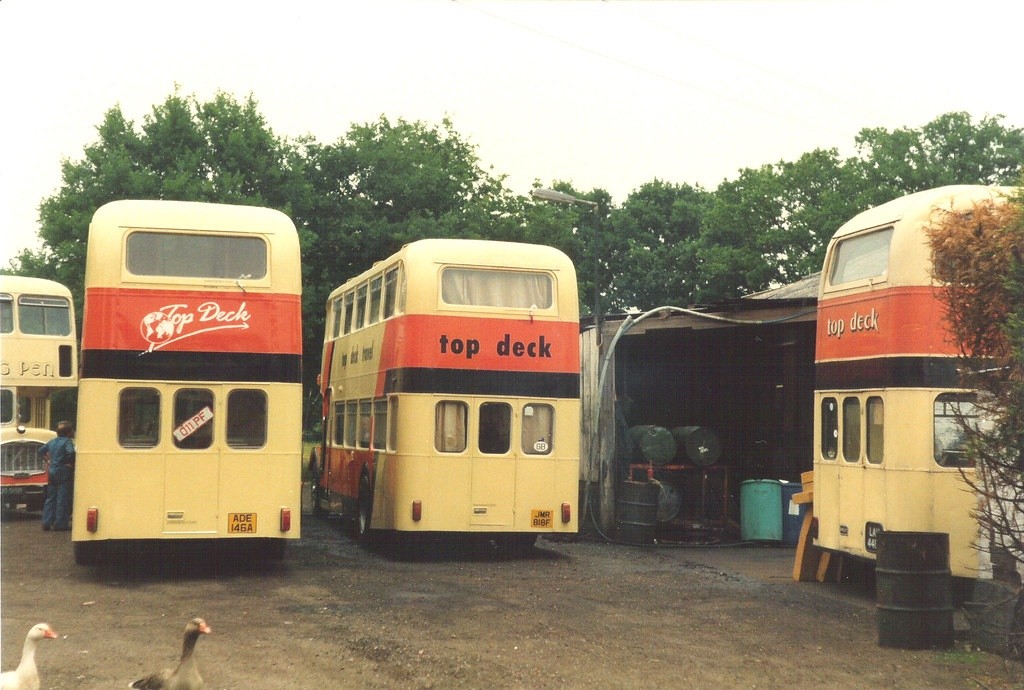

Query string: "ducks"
[1, 622, 58, 690]
[129, 617, 213, 690]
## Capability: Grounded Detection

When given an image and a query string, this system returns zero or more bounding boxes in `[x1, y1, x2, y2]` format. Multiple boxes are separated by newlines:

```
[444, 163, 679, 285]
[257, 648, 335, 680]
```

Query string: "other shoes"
[42, 524, 50, 530]
[54, 526, 72, 531]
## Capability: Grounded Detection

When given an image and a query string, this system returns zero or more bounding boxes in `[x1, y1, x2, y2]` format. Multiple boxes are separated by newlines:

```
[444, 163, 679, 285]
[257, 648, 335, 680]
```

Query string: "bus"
[70, 199, 303, 564]
[311, 238, 582, 548]
[0, 275, 78, 511]
[810, 184, 1024, 578]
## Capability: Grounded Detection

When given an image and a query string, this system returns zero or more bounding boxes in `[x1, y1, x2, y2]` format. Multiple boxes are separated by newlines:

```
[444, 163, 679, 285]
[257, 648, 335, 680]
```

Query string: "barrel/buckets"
[876, 529, 956, 651]
[619, 480, 660, 544]
[738, 478, 805, 545]
[626, 424, 724, 467]
[658, 481, 690, 522]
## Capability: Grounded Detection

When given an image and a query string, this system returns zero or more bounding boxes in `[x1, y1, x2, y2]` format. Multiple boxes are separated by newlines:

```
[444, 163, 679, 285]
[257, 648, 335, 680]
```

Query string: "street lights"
[533, 187, 602, 353]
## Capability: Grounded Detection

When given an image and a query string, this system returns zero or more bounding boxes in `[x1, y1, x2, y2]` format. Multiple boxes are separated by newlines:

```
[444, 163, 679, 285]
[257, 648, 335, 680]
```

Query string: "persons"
[36, 420, 75, 531]
